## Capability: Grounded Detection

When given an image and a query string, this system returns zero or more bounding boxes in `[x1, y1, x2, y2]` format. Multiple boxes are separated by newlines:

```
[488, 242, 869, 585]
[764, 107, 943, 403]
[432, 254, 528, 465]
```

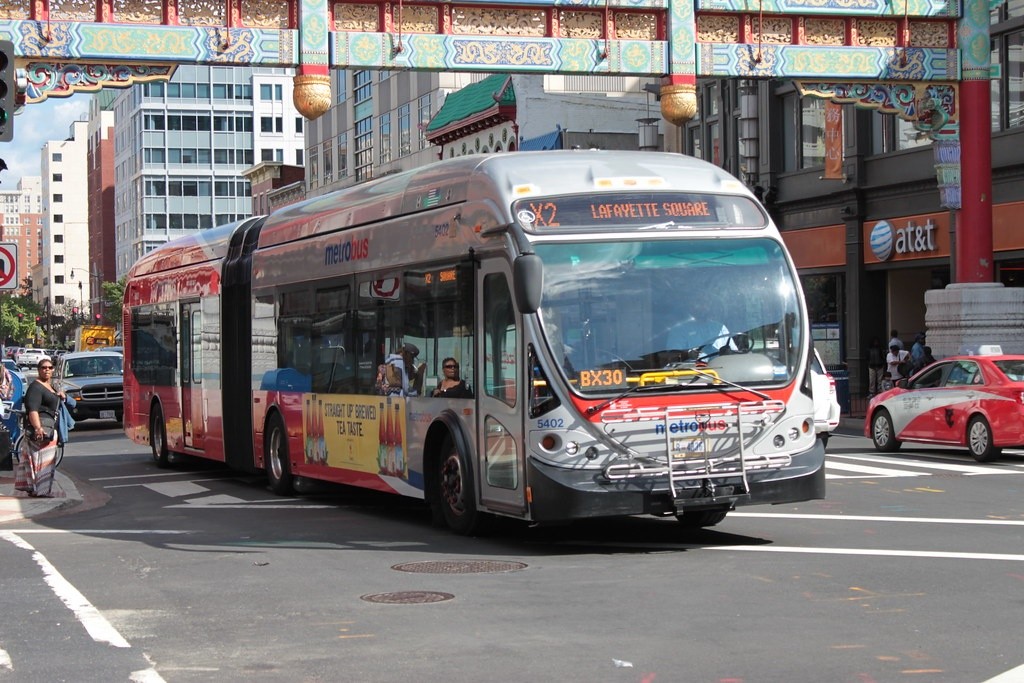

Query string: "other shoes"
[28, 492, 55, 498]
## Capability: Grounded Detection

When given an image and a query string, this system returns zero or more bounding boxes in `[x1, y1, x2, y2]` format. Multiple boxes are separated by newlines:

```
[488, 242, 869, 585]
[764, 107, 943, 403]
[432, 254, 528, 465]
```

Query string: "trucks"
[74, 325, 116, 352]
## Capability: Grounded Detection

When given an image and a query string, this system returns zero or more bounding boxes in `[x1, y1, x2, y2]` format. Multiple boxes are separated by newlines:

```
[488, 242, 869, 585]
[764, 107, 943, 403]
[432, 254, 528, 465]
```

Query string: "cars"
[2, 359, 29, 396]
[864, 343, 1024, 463]
[92, 347, 123, 367]
[5, 347, 69, 364]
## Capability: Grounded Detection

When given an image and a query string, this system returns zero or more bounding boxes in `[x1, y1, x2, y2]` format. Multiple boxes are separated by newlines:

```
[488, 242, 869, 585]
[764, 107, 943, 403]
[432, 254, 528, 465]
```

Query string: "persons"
[886, 345, 910, 387]
[867, 329, 885, 399]
[382, 343, 426, 396]
[666, 293, 739, 363]
[911, 334, 925, 360]
[15, 359, 66, 498]
[430, 357, 462, 396]
[106, 360, 115, 370]
[881, 372, 893, 392]
[913, 346, 943, 387]
[889, 329, 903, 352]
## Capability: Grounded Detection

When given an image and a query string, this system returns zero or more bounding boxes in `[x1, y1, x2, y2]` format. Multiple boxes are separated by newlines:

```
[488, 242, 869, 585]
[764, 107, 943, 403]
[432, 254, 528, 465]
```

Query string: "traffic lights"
[96, 314, 101, 325]
[18, 314, 22, 323]
[36, 317, 40, 326]
[73, 307, 78, 320]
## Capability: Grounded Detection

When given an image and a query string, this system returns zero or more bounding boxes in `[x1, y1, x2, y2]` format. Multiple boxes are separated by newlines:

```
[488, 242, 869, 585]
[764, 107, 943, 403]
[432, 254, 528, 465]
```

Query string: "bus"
[121, 152, 826, 535]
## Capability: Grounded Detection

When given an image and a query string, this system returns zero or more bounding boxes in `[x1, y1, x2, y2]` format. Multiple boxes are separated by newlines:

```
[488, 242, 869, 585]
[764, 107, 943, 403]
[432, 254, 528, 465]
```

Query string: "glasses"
[442, 365, 459, 369]
[39, 365, 54, 370]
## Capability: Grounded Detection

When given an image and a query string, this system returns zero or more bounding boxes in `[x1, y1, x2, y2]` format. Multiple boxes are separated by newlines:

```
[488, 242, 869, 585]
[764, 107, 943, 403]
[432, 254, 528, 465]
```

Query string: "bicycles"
[0, 408, 64, 472]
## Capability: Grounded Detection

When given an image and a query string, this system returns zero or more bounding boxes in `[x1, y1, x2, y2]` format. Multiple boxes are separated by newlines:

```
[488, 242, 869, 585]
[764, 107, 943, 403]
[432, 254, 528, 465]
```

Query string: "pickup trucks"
[52, 350, 125, 425]
[16, 350, 51, 370]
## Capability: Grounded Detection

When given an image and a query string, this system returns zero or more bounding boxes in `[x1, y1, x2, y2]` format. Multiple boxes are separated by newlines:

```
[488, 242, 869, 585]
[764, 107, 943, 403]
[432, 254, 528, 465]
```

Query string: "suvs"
[747, 341, 840, 444]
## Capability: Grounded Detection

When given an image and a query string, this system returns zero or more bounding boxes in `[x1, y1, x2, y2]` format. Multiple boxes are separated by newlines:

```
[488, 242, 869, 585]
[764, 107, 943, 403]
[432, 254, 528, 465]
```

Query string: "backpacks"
[375, 358, 403, 396]
[0, 362, 15, 401]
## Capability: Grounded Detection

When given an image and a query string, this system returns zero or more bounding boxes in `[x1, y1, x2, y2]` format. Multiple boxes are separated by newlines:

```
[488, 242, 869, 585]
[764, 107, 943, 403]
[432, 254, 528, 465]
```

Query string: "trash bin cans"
[825, 364, 850, 415]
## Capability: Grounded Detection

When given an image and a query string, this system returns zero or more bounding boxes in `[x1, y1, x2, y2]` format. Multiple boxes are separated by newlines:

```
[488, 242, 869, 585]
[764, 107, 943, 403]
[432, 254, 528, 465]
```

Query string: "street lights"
[27, 287, 40, 308]
[70, 268, 102, 321]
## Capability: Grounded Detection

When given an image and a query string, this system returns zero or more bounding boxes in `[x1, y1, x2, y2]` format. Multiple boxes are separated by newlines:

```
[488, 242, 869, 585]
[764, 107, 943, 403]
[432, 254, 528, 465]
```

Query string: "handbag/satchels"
[25, 416, 56, 442]
[897, 354, 915, 376]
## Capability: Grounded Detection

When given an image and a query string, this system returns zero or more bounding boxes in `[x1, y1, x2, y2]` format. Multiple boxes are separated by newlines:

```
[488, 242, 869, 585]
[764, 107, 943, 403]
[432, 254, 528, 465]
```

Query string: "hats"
[890, 345, 900, 351]
[403, 343, 420, 360]
[915, 333, 924, 340]
